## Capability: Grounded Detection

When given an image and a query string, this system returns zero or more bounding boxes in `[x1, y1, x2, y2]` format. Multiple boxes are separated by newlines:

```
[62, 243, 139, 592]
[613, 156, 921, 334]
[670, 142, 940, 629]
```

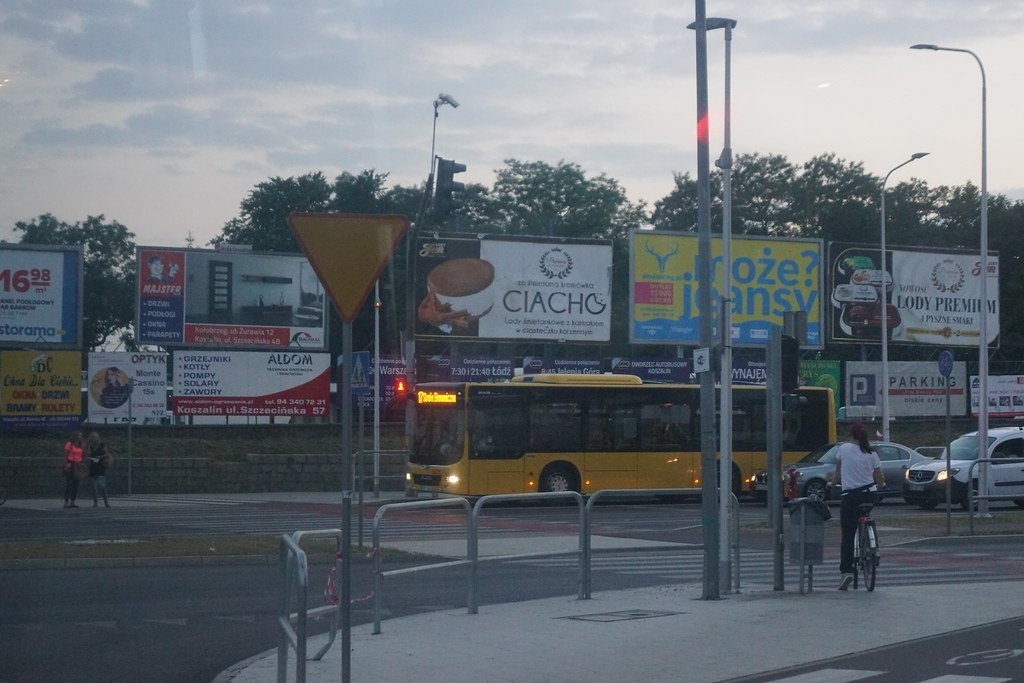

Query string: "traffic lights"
[434, 156, 466, 219]
[392, 376, 408, 401]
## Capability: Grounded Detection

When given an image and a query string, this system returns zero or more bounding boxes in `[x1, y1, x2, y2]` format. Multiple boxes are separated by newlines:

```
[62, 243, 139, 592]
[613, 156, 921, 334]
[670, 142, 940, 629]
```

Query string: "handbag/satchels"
[100, 453, 114, 468]
[69, 461, 89, 482]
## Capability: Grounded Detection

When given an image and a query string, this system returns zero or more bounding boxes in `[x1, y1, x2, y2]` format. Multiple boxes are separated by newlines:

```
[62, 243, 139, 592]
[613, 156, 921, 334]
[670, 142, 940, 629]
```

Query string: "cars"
[750, 441, 947, 507]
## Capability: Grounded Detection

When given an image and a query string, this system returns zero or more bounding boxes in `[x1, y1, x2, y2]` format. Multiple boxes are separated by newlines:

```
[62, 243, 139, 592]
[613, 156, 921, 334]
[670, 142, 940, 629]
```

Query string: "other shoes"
[874, 552, 881, 566]
[69, 505, 80, 508]
[838, 572, 854, 591]
[104, 503, 112, 509]
[63, 505, 72, 509]
[92, 502, 99, 509]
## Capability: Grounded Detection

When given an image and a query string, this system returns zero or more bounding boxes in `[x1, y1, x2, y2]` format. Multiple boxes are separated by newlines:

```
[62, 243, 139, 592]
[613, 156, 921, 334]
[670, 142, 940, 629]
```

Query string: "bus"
[405, 374, 837, 498]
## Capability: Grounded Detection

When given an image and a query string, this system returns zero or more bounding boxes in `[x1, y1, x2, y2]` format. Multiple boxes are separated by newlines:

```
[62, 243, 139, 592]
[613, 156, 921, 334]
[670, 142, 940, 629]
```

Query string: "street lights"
[911, 40, 988, 522]
[879, 152, 933, 442]
[687, 17, 739, 597]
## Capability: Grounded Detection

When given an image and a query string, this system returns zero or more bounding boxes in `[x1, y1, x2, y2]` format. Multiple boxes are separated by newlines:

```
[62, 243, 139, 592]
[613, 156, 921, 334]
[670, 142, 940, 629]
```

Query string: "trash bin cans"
[788, 498, 825, 565]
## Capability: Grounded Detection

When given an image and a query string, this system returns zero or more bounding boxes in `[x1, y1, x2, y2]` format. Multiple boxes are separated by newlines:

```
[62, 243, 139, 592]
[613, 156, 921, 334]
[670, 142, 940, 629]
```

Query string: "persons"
[830, 423, 885, 590]
[100, 367, 129, 409]
[83, 432, 111, 508]
[64, 431, 84, 508]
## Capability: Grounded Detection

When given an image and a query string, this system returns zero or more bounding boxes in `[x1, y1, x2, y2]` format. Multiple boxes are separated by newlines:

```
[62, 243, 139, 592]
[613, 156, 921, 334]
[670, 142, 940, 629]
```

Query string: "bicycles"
[827, 484, 881, 593]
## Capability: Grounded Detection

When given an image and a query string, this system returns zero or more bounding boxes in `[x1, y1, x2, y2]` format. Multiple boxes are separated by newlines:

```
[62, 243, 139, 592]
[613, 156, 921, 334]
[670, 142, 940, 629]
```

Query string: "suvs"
[903, 427, 1024, 512]
